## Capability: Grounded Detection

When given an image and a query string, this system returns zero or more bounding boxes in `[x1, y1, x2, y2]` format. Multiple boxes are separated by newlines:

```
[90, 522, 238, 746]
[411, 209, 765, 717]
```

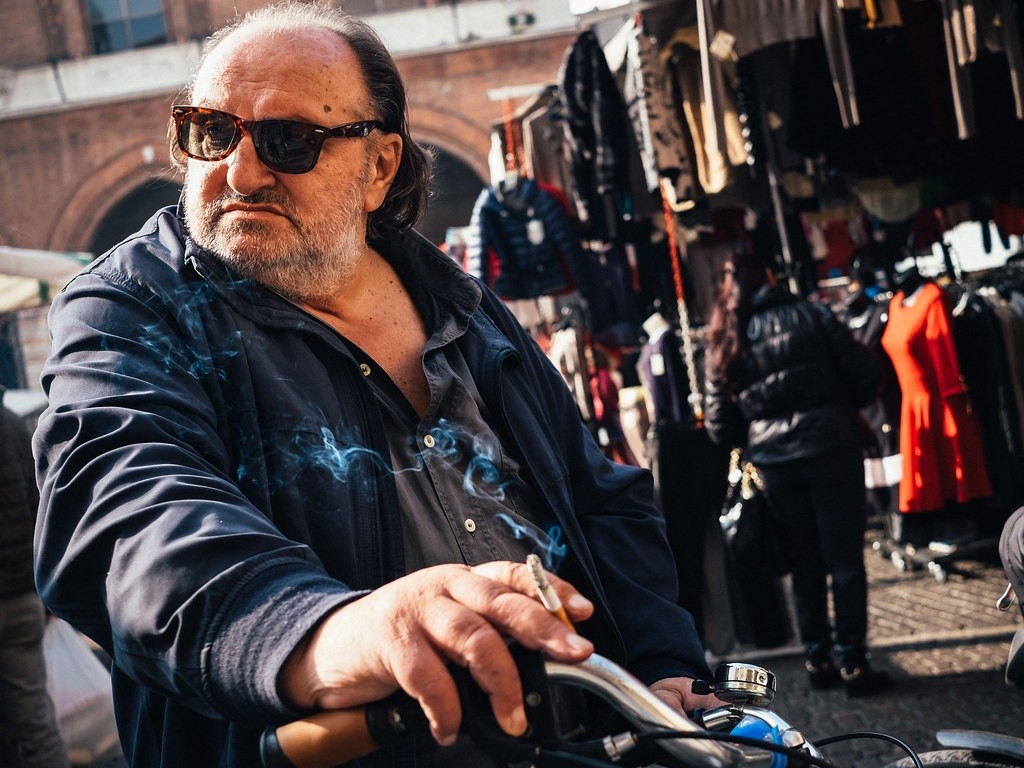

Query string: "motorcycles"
[259, 645, 923, 768]
[866, 583, 1024, 768]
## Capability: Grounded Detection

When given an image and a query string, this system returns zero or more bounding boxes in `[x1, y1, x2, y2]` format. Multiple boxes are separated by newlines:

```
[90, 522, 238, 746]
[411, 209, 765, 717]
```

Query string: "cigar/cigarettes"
[526, 554, 576, 635]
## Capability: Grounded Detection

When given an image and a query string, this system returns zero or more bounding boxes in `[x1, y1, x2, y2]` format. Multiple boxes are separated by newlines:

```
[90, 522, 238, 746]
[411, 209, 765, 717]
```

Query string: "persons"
[881, 266, 994, 513]
[0, 386, 71, 768]
[704, 252, 889, 693]
[31, 2, 731, 768]
[635, 312, 691, 423]
[585, 346, 630, 466]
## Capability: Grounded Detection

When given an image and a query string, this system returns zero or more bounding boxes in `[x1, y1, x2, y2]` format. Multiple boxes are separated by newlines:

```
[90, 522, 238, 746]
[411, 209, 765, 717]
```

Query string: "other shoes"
[805, 654, 887, 698]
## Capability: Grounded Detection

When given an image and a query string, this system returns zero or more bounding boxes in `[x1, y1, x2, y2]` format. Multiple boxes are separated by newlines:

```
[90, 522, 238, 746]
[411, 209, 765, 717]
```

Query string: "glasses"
[172, 104, 389, 174]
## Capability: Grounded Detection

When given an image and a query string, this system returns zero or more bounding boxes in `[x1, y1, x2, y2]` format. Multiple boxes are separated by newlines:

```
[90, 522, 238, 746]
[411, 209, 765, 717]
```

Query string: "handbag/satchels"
[718, 490, 793, 576]
